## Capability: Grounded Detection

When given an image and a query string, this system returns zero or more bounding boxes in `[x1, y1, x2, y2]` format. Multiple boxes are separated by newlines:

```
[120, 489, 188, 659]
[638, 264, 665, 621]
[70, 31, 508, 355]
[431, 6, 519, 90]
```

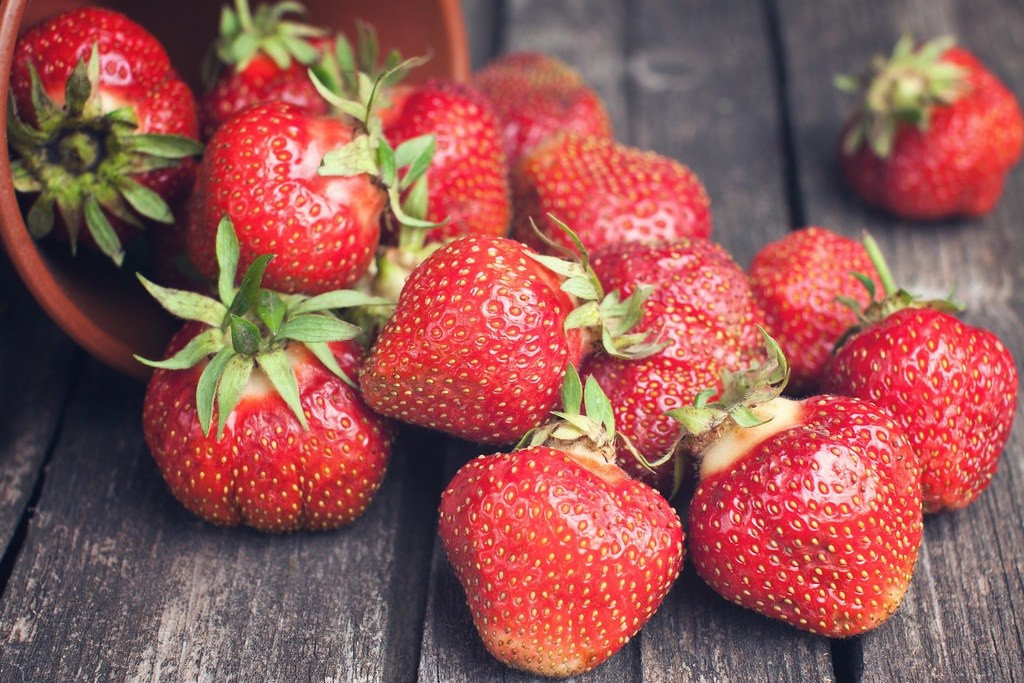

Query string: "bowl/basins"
[0, 0, 472, 381]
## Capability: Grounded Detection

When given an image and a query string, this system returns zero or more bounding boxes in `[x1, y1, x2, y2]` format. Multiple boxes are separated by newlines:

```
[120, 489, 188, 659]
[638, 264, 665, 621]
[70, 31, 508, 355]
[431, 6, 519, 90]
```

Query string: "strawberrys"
[4, 1, 1024, 679]
